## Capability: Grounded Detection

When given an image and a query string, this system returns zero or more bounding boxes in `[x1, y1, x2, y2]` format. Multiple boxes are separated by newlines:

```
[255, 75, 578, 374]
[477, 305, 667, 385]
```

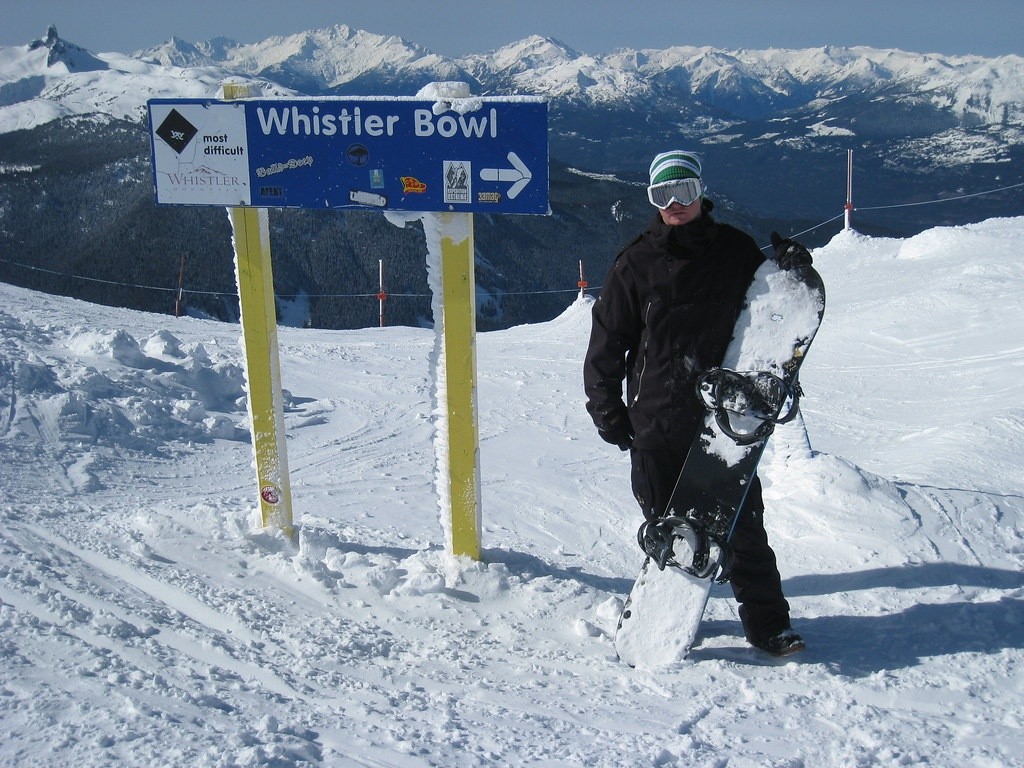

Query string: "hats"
[646, 149, 702, 186]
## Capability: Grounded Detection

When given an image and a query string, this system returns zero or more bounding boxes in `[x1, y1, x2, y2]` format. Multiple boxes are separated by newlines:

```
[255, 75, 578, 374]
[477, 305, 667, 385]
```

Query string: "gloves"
[770, 231, 814, 271]
[597, 407, 634, 452]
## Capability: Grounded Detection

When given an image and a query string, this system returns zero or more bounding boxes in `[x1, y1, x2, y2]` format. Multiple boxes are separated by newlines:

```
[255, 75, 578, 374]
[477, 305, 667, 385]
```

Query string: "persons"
[579, 146, 814, 658]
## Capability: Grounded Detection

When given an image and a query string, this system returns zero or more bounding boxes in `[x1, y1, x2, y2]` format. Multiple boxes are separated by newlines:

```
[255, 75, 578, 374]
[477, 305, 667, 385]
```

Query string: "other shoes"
[748, 625, 806, 656]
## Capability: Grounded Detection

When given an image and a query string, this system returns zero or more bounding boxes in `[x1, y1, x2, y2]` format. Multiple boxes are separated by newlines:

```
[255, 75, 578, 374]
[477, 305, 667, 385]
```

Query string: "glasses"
[646, 178, 701, 210]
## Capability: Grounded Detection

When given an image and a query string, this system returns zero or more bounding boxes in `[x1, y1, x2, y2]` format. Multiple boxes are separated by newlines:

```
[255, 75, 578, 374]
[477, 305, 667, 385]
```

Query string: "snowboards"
[614, 257, 825, 669]
